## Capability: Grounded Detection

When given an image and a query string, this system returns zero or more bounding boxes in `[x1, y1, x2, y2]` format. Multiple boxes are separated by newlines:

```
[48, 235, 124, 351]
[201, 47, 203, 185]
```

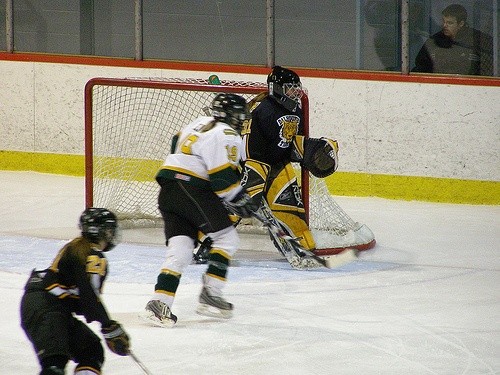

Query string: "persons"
[192, 66, 337, 268]
[139, 95, 251, 327]
[20, 207, 130, 375]
[413, 5, 494, 76]
[362, 1, 453, 73]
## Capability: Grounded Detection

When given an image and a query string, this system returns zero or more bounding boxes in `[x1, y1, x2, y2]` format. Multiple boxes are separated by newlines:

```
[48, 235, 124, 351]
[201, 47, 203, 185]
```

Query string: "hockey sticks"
[201, 106, 334, 274]
[250, 209, 359, 269]
[128, 349, 154, 375]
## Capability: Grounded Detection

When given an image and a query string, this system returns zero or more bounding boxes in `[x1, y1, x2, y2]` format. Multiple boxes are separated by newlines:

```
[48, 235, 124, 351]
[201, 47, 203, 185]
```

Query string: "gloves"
[230, 187, 259, 219]
[101, 320, 130, 356]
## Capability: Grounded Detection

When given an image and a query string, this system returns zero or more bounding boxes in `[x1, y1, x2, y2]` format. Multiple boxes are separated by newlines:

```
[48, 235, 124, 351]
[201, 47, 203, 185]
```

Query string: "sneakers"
[142, 300, 178, 329]
[195, 286, 233, 319]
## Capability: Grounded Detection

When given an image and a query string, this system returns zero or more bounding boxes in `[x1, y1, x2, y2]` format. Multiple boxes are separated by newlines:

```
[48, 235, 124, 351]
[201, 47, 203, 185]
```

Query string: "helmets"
[79, 208, 121, 252]
[267, 66, 303, 113]
[212, 94, 247, 120]
